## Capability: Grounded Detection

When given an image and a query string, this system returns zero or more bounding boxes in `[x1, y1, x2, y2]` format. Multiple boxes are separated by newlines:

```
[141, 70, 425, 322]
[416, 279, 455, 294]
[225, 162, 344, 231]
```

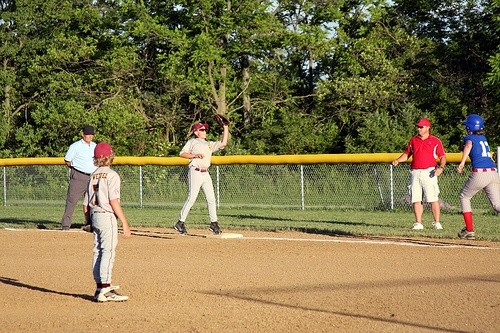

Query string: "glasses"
[418, 126, 424, 129]
[199, 129, 208, 132]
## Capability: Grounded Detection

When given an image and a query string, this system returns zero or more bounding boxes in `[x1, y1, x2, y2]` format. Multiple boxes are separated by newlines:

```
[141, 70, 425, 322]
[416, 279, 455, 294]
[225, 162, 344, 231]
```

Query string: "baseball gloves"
[213, 114, 232, 129]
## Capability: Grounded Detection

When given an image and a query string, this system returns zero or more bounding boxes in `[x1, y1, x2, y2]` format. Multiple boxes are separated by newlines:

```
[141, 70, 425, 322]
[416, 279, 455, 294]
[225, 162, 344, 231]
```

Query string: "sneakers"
[93, 285, 129, 302]
[173, 220, 188, 234]
[457, 227, 476, 240]
[208, 222, 222, 234]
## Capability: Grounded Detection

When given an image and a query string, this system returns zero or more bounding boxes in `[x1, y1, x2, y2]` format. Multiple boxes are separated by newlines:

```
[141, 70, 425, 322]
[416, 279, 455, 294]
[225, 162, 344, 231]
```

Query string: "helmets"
[461, 114, 485, 131]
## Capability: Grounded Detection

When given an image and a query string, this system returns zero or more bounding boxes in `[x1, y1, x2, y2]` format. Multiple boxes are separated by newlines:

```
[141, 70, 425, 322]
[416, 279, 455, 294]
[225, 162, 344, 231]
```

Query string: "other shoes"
[432, 221, 444, 230]
[62, 226, 70, 231]
[412, 222, 423, 230]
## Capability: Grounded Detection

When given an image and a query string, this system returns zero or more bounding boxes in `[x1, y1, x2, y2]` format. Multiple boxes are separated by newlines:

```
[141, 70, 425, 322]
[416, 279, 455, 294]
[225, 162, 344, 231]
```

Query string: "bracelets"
[437, 166, 445, 171]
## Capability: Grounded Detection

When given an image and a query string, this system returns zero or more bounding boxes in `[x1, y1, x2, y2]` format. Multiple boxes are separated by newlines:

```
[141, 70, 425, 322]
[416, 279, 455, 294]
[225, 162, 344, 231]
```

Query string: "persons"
[60, 125, 98, 231]
[391, 120, 446, 229]
[174, 114, 229, 234]
[82, 142, 131, 303]
[456, 114, 500, 240]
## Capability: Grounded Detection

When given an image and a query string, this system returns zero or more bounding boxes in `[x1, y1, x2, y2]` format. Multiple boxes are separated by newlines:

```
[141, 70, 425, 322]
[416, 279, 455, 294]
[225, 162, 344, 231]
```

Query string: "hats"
[94, 141, 112, 159]
[414, 118, 431, 127]
[83, 125, 96, 135]
[191, 123, 208, 132]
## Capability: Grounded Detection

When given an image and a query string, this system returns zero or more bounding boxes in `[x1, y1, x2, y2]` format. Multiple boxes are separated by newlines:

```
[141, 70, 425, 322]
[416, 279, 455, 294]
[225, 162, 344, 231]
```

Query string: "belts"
[195, 168, 206, 172]
[71, 166, 91, 176]
[94, 210, 112, 213]
[472, 167, 496, 172]
